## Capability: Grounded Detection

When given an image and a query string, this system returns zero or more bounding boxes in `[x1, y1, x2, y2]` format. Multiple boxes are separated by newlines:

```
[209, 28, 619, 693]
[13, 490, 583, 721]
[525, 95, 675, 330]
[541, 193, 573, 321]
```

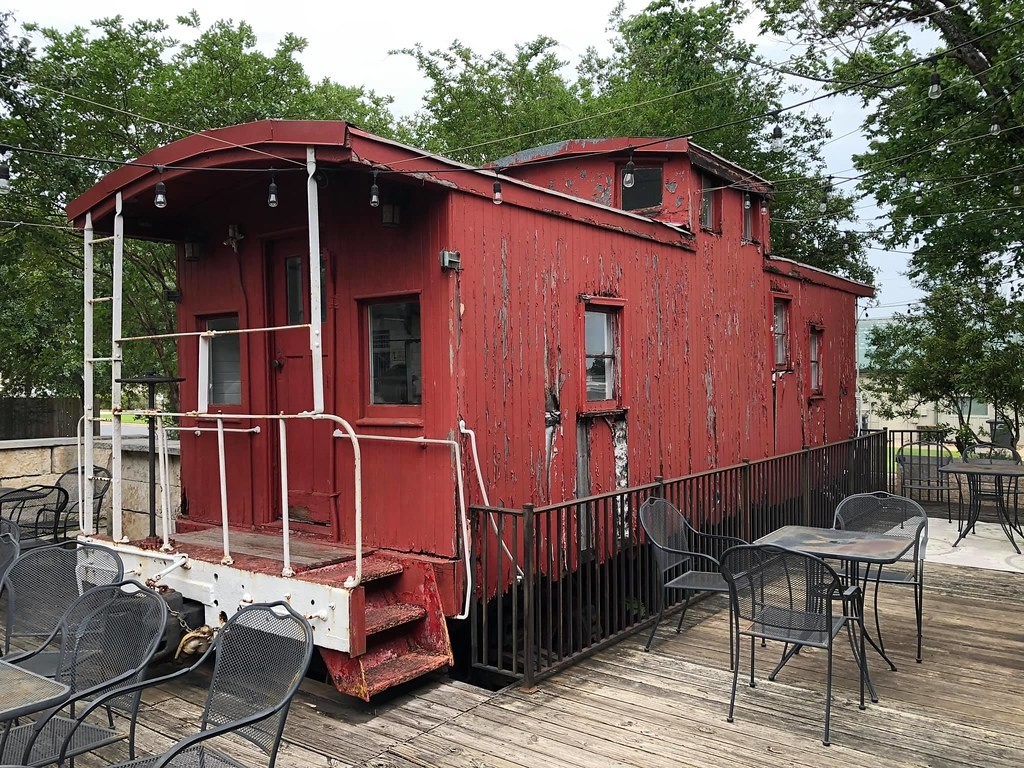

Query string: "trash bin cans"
[986, 419, 1013, 448]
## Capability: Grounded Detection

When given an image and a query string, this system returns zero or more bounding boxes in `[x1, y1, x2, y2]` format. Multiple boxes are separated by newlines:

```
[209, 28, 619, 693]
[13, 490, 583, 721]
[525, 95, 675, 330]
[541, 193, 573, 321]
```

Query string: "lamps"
[380, 198, 403, 227]
[185, 237, 200, 261]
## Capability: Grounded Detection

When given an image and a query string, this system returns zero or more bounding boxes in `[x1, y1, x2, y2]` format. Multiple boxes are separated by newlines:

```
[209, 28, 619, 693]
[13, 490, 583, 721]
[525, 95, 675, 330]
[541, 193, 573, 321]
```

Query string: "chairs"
[963, 442, 1024, 533]
[896, 442, 960, 528]
[0, 465, 313, 768]
[719, 541, 867, 747]
[833, 491, 929, 662]
[639, 495, 766, 672]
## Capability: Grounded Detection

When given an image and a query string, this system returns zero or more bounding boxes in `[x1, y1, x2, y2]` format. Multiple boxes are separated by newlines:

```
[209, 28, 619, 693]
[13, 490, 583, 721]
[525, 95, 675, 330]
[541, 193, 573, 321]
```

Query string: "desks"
[752, 524, 916, 702]
[1, 660, 71, 726]
[937, 462, 1024, 554]
[1, 488, 45, 534]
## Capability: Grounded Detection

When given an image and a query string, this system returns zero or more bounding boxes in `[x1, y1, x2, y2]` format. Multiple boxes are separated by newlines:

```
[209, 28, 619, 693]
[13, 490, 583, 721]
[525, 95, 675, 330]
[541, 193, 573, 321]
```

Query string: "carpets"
[883, 516, 1024, 574]
